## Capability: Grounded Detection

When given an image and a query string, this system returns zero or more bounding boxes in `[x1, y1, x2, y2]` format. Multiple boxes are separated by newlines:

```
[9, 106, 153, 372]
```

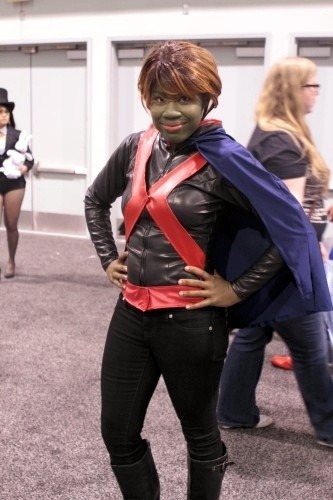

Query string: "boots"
[111, 438, 160, 500]
[187, 440, 235, 500]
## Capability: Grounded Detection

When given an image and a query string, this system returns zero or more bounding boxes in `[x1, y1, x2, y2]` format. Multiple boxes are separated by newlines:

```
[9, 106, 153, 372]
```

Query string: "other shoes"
[4, 262, 15, 278]
[219, 414, 272, 429]
[271, 354, 294, 370]
[313, 432, 333, 447]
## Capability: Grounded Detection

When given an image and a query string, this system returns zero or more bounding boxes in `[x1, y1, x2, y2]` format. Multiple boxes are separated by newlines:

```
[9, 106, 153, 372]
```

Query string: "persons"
[84, 40, 333, 500]
[0, 87, 35, 278]
[215, 56, 333, 445]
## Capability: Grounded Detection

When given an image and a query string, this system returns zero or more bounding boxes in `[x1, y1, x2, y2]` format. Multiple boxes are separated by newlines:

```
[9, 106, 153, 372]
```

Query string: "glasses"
[300, 83, 321, 89]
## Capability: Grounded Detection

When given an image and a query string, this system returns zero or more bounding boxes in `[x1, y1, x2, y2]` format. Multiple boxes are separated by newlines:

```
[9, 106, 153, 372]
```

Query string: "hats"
[0, 88, 15, 109]
